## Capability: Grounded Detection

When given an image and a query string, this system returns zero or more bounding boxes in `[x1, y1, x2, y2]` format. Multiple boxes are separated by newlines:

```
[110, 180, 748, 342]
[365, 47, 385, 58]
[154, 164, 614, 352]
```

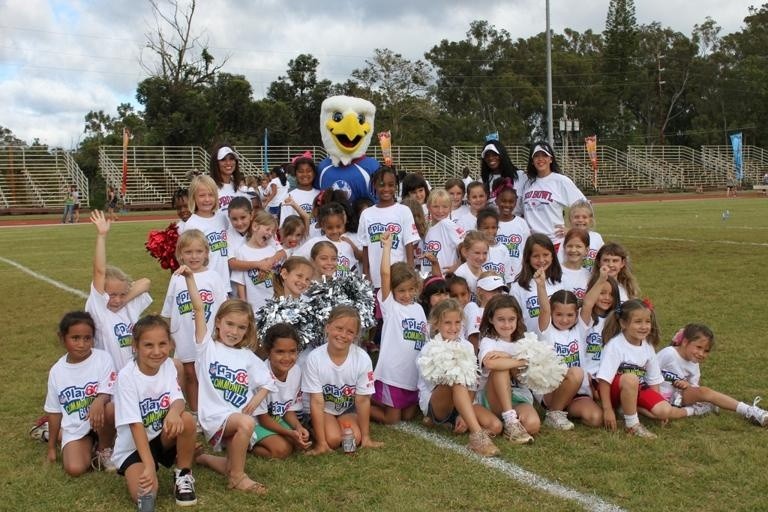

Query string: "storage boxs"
[511, 159, 527, 171]
[459, 161, 480, 178]
[0, 166, 88, 214]
[106, 169, 219, 209]
[239, 163, 284, 175]
[376, 160, 461, 186]
[555, 144, 768, 191]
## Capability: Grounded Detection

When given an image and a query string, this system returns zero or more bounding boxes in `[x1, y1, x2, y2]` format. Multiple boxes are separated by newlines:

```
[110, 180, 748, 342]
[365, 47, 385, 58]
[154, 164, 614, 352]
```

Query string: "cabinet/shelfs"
[340, 422, 356, 458]
[137, 487, 155, 512]
[669, 387, 682, 407]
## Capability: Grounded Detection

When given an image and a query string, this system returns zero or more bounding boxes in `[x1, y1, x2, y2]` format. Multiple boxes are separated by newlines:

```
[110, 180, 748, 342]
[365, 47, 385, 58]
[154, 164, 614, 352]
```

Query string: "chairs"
[691, 401, 720, 417]
[623, 423, 657, 442]
[172, 467, 197, 508]
[29, 421, 49, 443]
[94, 447, 117, 475]
[744, 405, 768, 428]
[502, 420, 534, 446]
[542, 410, 574, 432]
[467, 430, 501, 458]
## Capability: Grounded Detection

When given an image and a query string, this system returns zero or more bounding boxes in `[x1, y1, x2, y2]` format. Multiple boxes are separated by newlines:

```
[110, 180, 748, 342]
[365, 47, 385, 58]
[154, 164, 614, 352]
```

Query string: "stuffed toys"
[313, 95, 383, 208]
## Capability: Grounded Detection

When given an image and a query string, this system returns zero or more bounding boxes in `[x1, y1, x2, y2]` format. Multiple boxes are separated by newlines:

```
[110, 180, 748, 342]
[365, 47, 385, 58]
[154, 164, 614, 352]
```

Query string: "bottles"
[480, 143, 500, 158]
[476, 276, 510, 294]
[530, 144, 553, 159]
[216, 146, 239, 160]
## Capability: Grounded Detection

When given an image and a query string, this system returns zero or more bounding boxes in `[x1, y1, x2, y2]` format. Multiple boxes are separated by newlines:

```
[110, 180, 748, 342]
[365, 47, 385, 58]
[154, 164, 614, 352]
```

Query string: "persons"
[84, 208, 153, 375]
[62, 185, 80, 224]
[107, 186, 119, 221]
[28, 310, 116, 478]
[161, 140, 640, 459]
[109, 314, 196, 512]
[174, 264, 279, 498]
[594, 297, 720, 439]
[653, 323, 766, 429]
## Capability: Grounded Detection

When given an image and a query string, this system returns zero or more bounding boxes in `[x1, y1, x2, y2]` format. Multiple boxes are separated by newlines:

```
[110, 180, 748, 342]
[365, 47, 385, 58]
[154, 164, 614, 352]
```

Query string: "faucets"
[174, 443, 204, 462]
[224, 474, 268, 497]
